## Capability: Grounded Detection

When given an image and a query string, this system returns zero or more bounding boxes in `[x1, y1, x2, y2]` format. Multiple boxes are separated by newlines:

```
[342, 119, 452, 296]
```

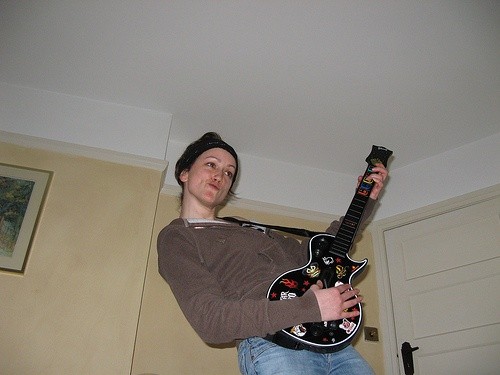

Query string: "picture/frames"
[0, 162, 53, 273]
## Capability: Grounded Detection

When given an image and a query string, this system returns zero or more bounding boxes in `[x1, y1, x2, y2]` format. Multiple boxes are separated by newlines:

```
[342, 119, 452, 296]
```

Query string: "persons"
[157, 130, 389, 375]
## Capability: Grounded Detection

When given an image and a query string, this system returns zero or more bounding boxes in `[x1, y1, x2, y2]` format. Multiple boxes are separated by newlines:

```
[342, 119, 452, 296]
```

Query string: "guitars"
[267, 144, 393, 353]
